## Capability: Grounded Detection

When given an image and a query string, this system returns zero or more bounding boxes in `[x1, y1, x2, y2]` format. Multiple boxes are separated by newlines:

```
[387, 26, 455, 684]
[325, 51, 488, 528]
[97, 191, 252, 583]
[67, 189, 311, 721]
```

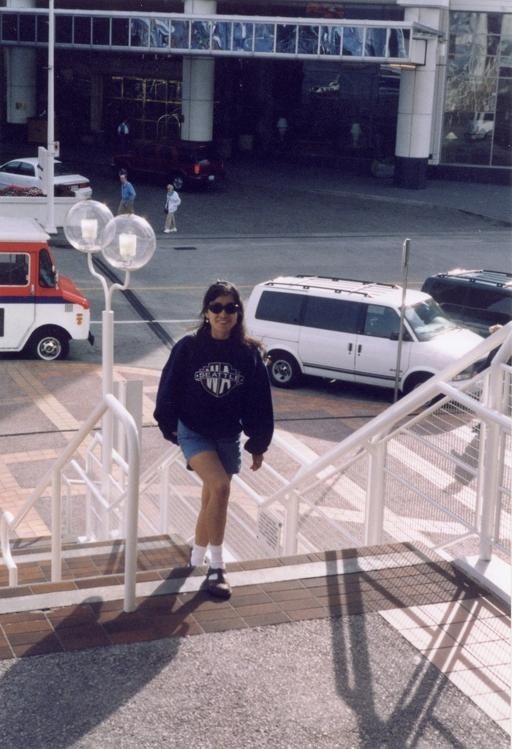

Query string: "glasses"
[207, 302, 239, 313]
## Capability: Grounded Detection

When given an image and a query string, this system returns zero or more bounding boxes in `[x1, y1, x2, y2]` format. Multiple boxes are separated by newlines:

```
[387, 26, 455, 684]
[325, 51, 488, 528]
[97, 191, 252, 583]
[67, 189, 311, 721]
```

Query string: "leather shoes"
[185, 560, 232, 598]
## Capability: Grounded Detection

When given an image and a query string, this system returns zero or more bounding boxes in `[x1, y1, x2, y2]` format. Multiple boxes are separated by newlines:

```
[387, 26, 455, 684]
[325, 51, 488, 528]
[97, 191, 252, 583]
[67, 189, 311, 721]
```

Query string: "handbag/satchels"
[163, 208, 168, 212]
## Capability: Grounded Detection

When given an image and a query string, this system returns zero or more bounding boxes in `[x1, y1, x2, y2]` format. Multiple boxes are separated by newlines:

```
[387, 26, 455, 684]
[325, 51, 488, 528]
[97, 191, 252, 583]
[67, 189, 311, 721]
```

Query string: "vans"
[463, 111, 494, 143]
[243, 268, 511, 405]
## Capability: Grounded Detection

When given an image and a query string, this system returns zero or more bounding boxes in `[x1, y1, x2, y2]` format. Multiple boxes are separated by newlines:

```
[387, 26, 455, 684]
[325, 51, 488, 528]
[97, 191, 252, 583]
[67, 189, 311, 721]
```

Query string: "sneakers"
[164, 228, 178, 233]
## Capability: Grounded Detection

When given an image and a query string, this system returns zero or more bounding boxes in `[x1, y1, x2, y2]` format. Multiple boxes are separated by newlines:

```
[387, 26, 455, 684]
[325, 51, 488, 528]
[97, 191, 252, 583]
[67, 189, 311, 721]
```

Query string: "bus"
[0, 217, 95, 360]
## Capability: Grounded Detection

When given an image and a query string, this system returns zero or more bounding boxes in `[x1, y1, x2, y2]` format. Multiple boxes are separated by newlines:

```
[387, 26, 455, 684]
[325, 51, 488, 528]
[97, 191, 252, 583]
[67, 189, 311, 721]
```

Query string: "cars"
[93, 135, 233, 193]
[0, 157, 93, 198]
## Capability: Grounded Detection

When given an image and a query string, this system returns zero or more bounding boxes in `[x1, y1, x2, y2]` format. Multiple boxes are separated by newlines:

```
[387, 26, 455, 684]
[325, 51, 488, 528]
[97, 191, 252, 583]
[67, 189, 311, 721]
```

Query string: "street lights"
[64, 200, 156, 534]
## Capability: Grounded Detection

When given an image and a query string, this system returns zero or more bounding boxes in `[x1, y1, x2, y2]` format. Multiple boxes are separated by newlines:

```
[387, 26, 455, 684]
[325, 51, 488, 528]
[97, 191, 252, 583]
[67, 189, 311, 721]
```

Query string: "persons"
[117, 172, 136, 217]
[117, 116, 130, 153]
[152, 279, 275, 599]
[163, 183, 182, 234]
